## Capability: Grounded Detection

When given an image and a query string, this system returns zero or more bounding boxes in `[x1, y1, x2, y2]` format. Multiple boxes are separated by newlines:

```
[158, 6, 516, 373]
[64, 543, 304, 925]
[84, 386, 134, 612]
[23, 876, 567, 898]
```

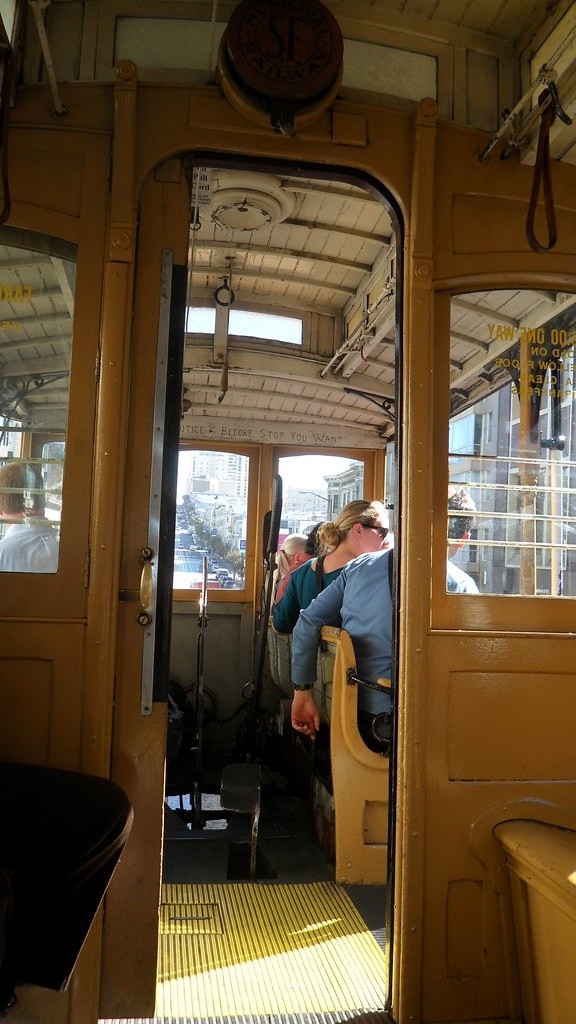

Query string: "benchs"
[259, 614, 389, 887]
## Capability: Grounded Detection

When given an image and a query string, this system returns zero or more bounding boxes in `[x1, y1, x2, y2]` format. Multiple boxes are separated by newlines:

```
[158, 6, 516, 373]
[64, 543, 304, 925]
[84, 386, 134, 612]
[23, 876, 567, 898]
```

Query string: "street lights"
[298, 489, 332, 522]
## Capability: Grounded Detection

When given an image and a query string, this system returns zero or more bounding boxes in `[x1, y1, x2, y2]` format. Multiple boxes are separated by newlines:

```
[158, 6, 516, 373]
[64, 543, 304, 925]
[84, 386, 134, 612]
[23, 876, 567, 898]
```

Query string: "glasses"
[347, 521, 389, 540]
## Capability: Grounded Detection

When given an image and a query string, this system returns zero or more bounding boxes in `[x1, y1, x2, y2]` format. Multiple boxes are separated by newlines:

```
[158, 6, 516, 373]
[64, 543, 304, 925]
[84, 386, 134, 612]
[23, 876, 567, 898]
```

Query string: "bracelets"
[293, 683, 314, 691]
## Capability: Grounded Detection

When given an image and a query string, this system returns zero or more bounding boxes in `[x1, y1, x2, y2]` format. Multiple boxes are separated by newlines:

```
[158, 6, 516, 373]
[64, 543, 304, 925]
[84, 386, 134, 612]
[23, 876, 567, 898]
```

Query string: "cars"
[174, 509, 236, 590]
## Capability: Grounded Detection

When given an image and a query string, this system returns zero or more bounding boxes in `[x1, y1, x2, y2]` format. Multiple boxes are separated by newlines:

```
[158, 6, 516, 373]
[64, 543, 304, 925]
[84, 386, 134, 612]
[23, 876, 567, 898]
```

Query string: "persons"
[0, 760, 134, 1006]
[270, 493, 480, 755]
[0, 462, 59, 574]
